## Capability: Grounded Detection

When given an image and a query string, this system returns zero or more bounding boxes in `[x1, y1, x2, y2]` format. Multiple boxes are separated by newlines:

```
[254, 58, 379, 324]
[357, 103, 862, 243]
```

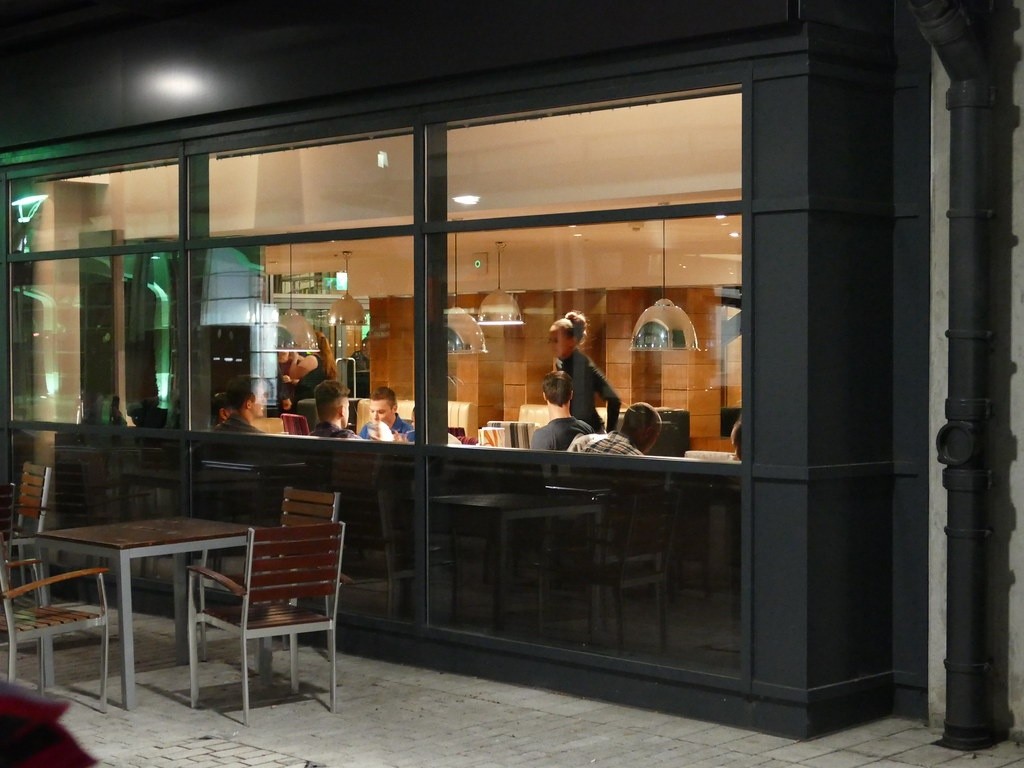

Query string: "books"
[274, 269, 337, 295]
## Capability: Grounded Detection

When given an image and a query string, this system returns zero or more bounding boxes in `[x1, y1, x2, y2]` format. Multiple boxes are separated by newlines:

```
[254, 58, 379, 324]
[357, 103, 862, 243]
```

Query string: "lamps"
[337, 251, 364, 324]
[477, 241, 523, 326]
[276, 243, 318, 353]
[629, 219, 699, 349]
[446, 232, 485, 352]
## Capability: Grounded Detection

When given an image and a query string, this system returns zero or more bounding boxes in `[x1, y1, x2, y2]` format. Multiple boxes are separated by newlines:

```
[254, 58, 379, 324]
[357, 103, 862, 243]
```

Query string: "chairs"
[10, 462, 52, 606]
[186, 522, 343, 724]
[281, 414, 308, 434]
[0, 532, 108, 713]
[201, 488, 339, 694]
[251, 418, 283, 433]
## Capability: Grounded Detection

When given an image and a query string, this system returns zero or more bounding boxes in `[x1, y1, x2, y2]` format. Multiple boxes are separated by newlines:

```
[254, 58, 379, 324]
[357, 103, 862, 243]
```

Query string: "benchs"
[520, 405, 606, 429]
[356, 398, 477, 445]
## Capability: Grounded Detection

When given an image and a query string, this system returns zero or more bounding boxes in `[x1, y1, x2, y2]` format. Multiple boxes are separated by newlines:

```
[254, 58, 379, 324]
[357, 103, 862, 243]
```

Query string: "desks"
[39, 517, 256, 712]
[297, 398, 356, 430]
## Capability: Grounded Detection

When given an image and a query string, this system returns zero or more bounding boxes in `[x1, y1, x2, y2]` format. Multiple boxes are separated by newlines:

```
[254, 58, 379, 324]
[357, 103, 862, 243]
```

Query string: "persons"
[530, 371, 595, 451]
[277, 352, 306, 414]
[567, 400, 663, 455]
[346, 337, 370, 399]
[210, 376, 267, 435]
[289, 330, 338, 405]
[360, 385, 412, 442]
[731, 419, 742, 461]
[548, 312, 621, 436]
[411, 407, 463, 446]
[306, 381, 364, 440]
